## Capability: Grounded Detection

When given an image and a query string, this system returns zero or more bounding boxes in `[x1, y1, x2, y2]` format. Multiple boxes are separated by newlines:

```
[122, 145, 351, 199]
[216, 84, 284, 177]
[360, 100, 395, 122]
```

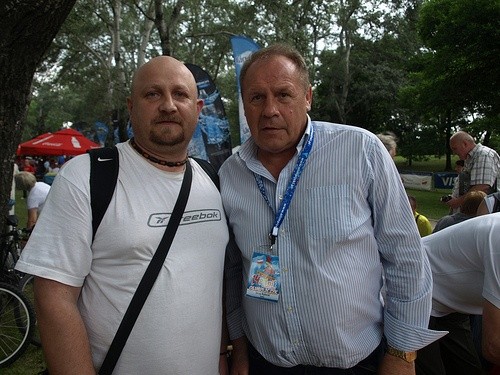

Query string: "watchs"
[387, 346, 417, 363]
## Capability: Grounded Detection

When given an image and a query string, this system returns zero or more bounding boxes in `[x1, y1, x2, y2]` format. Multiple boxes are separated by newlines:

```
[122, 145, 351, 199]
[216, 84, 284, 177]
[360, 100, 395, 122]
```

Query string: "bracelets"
[219, 350, 229, 356]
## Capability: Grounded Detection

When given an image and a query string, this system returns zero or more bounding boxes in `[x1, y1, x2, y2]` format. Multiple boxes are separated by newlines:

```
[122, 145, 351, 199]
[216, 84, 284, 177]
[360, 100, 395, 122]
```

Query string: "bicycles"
[0, 214, 42, 369]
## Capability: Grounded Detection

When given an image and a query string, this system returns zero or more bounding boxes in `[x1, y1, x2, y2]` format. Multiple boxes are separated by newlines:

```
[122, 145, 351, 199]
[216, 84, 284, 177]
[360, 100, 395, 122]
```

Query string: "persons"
[375, 132, 500, 375]
[14, 54, 230, 375]
[14, 158, 54, 231]
[217, 44, 451, 375]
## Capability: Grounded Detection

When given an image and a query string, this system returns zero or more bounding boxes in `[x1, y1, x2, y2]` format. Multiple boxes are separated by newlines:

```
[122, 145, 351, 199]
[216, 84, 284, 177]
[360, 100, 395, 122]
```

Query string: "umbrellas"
[17, 116, 102, 160]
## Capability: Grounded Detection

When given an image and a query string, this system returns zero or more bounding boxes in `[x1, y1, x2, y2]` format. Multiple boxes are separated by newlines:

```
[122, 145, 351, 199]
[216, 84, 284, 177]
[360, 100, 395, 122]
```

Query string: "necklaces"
[129, 137, 190, 167]
